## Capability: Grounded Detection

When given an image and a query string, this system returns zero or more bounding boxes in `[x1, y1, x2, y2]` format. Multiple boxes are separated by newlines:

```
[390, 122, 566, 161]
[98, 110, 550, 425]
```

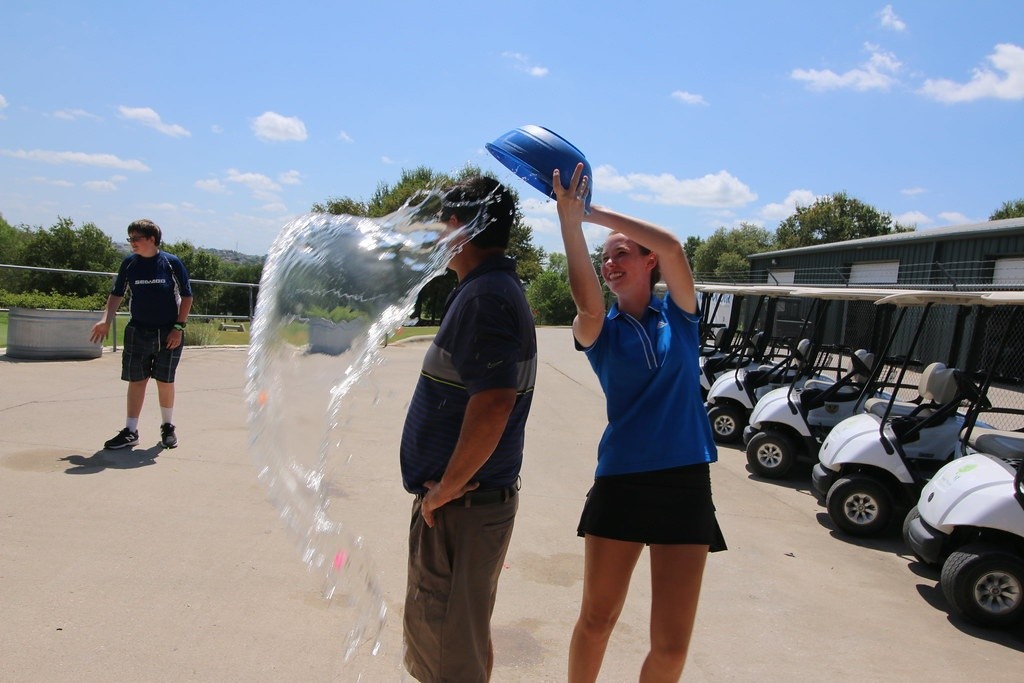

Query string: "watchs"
[175, 322, 187, 328]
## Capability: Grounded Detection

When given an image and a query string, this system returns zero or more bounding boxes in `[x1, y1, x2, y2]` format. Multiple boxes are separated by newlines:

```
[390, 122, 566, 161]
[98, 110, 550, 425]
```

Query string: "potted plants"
[305, 303, 370, 354]
[0, 287, 107, 360]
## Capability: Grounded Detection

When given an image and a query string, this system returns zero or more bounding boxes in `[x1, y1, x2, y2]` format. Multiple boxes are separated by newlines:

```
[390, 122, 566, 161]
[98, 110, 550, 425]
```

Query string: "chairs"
[758, 339, 809, 383]
[804, 349, 874, 402]
[708, 331, 765, 368]
[960, 426, 1024, 458]
[864, 362, 956, 419]
[697, 326, 727, 354]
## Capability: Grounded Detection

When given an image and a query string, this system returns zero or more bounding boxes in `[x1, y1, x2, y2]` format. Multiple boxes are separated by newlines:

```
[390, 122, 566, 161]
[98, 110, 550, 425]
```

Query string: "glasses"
[127, 236, 149, 242]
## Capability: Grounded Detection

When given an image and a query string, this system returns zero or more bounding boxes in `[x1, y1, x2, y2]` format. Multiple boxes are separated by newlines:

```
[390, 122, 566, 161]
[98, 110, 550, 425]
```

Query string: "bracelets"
[174, 325, 185, 332]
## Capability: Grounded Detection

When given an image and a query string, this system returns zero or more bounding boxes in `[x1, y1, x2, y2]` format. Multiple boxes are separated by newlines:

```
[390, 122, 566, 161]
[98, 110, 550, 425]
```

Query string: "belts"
[418, 481, 517, 505]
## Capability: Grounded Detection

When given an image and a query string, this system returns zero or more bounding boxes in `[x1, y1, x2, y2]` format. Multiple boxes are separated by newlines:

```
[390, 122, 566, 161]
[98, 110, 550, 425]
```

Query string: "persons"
[552, 161, 729, 683]
[89, 219, 192, 450]
[400, 176, 538, 683]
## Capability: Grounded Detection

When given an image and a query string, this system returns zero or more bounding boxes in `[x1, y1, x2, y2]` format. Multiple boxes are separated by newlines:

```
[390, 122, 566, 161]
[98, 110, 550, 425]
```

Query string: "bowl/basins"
[484, 125, 594, 216]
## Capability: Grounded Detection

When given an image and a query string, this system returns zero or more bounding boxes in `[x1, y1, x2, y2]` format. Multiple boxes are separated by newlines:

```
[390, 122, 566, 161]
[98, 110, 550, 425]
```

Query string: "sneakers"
[160, 423, 178, 448]
[104, 428, 139, 449]
